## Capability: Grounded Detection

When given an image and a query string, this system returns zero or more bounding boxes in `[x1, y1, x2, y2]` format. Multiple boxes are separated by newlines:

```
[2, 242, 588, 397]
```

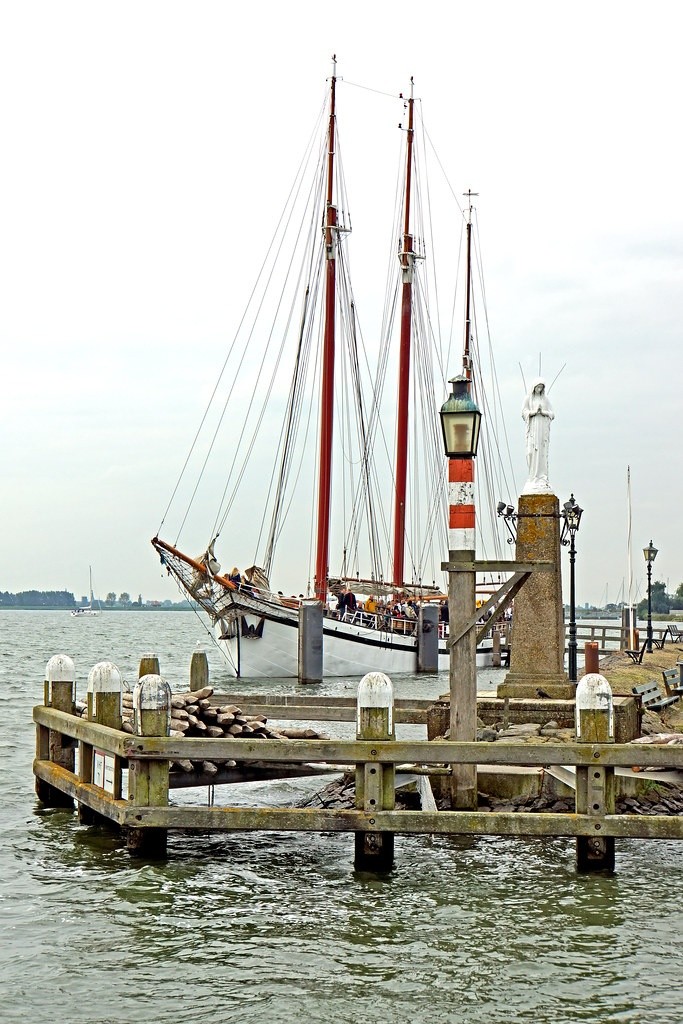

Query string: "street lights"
[564, 493, 584, 680]
[642, 539, 658, 652]
[437, 374, 482, 810]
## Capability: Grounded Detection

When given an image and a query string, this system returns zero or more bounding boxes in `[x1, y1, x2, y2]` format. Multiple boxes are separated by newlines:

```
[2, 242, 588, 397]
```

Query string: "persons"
[476, 598, 514, 622]
[338, 584, 448, 636]
[222, 566, 255, 597]
[521, 377, 555, 485]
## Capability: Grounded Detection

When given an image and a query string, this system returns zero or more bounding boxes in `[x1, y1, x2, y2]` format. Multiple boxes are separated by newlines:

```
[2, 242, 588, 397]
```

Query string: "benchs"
[662, 668, 683, 697]
[667, 625, 683, 644]
[625, 638, 649, 665]
[632, 679, 680, 711]
[652, 629, 668, 650]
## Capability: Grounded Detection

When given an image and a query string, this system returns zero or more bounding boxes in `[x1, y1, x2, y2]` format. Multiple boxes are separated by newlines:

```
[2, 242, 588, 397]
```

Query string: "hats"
[407, 600, 412, 604]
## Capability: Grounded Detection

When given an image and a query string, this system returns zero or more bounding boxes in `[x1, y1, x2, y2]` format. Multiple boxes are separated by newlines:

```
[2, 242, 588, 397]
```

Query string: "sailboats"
[152, 55, 518, 682]
[71, 565, 103, 617]
